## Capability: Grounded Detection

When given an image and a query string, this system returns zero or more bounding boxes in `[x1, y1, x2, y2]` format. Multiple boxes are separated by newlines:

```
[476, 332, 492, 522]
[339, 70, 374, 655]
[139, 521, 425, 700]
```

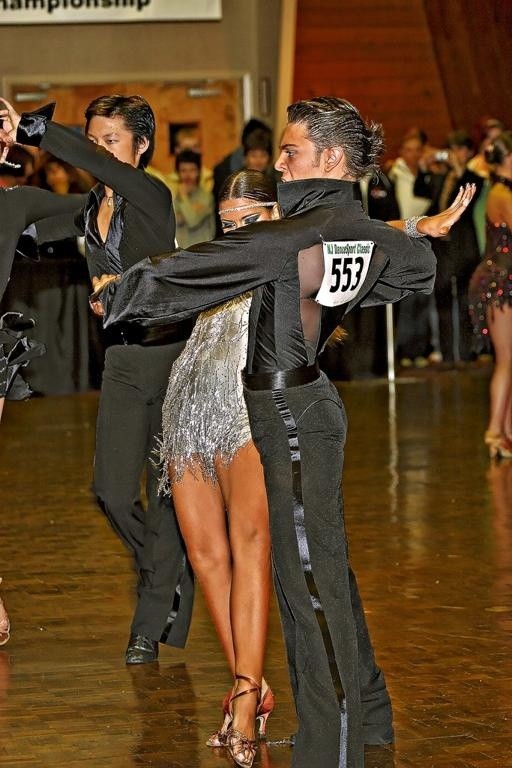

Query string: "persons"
[91, 170, 476, 768]
[0, 101, 88, 644]
[1, 93, 187, 666]
[92, 98, 438, 767]
[467, 131, 511, 459]
[16, 119, 504, 389]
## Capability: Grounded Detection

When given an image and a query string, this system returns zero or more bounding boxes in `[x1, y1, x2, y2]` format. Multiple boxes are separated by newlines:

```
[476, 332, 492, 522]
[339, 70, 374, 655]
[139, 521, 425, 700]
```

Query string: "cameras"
[435, 151, 449, 162]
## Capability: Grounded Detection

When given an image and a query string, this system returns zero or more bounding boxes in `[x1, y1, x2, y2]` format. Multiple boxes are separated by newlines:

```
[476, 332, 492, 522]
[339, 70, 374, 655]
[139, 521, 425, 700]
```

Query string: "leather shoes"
[125, 634, 160, 665]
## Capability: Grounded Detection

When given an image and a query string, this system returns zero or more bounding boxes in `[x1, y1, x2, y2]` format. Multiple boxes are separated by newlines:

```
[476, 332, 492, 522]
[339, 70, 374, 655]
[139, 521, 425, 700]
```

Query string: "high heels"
[485, 432, 511, 458]
[206, 673, 275, 767]
